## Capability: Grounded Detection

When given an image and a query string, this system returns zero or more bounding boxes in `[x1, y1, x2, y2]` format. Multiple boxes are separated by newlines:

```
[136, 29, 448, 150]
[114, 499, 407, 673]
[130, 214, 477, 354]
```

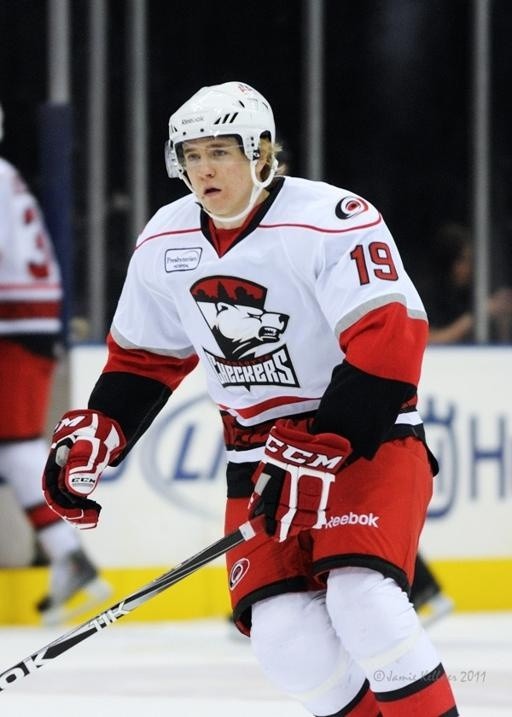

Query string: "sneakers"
[37, 549, 96, 611]
[410, 555, 440, 606]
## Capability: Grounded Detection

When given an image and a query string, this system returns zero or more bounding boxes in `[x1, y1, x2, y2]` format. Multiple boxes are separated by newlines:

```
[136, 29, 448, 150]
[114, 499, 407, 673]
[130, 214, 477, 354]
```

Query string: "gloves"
[44, 409, 126, 532]
[246, 418, 350, 543]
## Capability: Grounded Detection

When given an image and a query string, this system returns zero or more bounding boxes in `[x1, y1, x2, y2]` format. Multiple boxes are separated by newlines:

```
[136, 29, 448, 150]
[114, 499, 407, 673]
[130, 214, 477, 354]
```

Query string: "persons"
[411, 205, 510, 344]
[40, 78, 462, 715]
[0, 156, 100, 613]
[409, 550, 441, 611]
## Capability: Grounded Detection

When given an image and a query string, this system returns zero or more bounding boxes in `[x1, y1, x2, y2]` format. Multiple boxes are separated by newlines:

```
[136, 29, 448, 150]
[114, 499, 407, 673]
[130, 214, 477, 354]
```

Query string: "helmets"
[165, 82, 277, 221]
